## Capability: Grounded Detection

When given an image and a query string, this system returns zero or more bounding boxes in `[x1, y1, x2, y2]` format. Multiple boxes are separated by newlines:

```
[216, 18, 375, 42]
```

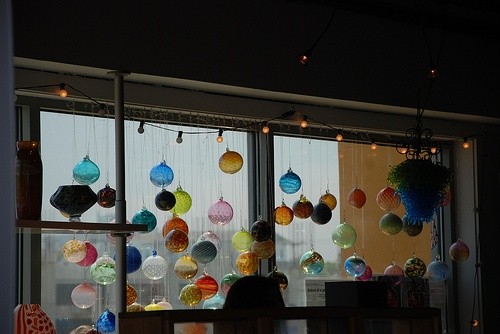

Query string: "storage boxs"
[325, 282, 388, 307]
[373, 275, 430, 306]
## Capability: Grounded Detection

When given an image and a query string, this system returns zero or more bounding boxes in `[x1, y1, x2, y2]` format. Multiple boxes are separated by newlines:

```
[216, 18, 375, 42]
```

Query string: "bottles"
[16, 140, 42, 221]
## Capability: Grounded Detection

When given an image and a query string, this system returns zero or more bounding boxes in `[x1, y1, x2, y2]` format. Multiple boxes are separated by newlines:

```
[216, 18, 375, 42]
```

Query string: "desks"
[119, 306, 443, 334]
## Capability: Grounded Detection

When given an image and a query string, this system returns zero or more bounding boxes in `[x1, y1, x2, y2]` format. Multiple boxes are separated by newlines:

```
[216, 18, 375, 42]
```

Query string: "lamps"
[297, 0, 338, 66]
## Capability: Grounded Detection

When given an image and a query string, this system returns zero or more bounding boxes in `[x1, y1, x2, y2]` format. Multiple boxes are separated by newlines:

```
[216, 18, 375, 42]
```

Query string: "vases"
[16, 141, 42, 220]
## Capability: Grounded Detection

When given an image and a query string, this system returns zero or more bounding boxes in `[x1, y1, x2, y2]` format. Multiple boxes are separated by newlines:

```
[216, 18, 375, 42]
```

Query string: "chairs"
[224, 276, 286, 307]
